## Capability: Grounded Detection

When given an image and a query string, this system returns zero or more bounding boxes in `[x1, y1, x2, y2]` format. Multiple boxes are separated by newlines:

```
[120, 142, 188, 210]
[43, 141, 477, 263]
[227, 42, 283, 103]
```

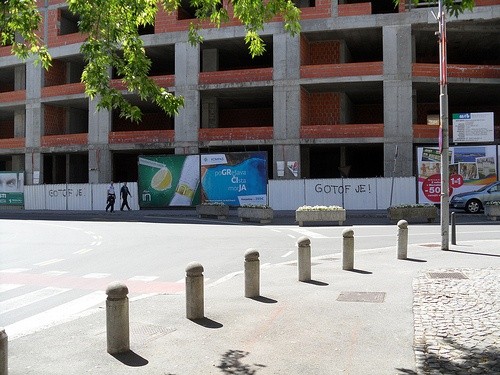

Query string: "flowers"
[486, 201, 500, 205]
[298, 205, 343, 211]
[392, 202, 436, 209]
[241, 203, 271, 209]
[201, 202, 224, 206]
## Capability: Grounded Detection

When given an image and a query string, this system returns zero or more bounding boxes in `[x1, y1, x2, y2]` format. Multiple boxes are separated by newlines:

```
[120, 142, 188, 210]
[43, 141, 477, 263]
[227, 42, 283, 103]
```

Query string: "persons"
[120, 182, 131, 211]
[106, 181, 116, 213]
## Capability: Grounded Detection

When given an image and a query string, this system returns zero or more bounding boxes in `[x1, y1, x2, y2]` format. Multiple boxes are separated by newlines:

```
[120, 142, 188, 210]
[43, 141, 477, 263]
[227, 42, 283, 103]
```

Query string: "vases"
[196, 205, 229, 215]
[238, 207, 273, 218]
[387, 208, 438, 219]
[484, 205, 500, 216]
[296, 209, 346, 221]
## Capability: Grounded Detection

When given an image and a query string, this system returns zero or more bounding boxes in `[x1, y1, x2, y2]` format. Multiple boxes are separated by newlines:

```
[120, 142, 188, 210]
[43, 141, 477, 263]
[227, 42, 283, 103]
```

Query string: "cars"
[450, 181, 500, 214]
